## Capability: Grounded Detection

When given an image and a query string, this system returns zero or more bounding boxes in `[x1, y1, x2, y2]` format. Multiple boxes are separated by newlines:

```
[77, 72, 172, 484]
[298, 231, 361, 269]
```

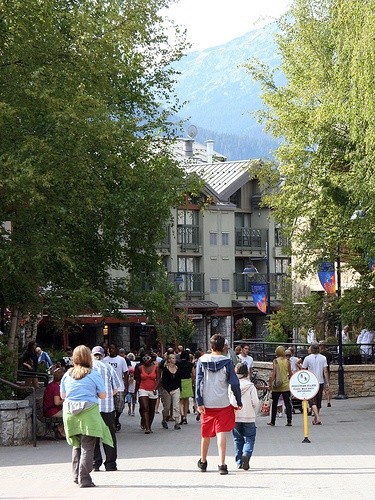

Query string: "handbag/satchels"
[260, 389, 271, 416]
[272, 359, 283, 390]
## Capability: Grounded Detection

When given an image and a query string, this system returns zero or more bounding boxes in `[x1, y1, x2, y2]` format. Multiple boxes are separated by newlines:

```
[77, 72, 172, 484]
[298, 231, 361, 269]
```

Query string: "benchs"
[35, 397, 63, 441]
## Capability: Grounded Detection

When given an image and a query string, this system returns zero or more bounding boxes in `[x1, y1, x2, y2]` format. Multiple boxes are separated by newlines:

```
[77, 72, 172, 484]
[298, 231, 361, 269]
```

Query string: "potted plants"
[235, 317, 253, 340]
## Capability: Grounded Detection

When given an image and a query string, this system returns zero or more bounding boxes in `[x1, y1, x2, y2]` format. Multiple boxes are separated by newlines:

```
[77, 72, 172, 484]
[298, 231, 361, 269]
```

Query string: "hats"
[318, 341, 328, 346]
[90, 345, 104, 357]
[224, 338, 229, 346]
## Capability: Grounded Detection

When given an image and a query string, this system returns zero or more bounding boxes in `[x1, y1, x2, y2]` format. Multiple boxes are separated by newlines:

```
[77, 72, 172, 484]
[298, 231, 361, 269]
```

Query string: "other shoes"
[140, 424, 145, 430]
[178, 415, 185, 425]
[237, 464, 242, 469]
[285, 422, 292, 426]
[312, 420, 316, 423]
[144, 429, 153, 434]
[128, 410, 131, 416]
[267, 422, 275, 426]
[327, 403, 331, 407]
[115, 423, 121, 431]
[196, 412, 201, 421]
[131, 412, 134, 416]
[185, 417, 189, 424]
[242, 456, 249, 470]
[313, 421, 322, 425]
[162, 420, 168, 429]
[174, 424, 181, 430]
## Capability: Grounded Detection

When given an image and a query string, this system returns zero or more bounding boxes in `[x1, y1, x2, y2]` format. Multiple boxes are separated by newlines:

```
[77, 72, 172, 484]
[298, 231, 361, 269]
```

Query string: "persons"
[35, 345, 54, 378]
[59, 344, 107, 488]
[318, 342, 332, 407]
[151, 344, 205, 421]
[194, 334, 243, 476]
[42, 361, 66, 440]
[227, 363, 263, 471]
[265, 346, 315, 418]
[221, 339, 258, 428]
[301, 344, 330, 425]
[91, 345, 122, 471]
[356, 325, 375, 365]
[338, 325, 351, 361]
[20, 341, 40, 390]
[101, 342, 154, 430]
[266, 345, 293, 427]
[101, 342, 128, 431]
[134, 350, 161, 434]
[174, 350, 194, 426]
[159, 353, 182, 430]
[61, 346, 74, 370]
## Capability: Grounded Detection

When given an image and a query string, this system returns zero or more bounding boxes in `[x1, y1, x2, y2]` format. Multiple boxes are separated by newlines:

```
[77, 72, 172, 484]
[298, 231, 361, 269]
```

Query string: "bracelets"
[153, 389, 156, 392]
[130, 375, 134, 377]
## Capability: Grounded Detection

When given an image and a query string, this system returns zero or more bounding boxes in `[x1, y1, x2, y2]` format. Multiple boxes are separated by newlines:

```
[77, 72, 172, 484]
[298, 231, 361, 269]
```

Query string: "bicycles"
[251, 369, 267, 399]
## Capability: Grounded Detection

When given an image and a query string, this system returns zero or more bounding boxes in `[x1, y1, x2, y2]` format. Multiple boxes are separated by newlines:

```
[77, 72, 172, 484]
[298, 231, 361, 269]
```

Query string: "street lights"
[174, 273, 184, 305]
[335, 210, 365, 399]
[241, 242, 270, 321]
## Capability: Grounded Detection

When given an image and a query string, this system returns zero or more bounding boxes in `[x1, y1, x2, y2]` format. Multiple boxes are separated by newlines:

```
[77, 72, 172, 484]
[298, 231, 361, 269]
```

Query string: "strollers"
[291, 396, 303, 414]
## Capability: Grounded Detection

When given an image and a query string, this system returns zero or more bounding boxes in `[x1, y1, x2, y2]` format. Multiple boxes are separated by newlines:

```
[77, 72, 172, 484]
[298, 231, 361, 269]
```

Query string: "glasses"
[141, 356, 152, 362]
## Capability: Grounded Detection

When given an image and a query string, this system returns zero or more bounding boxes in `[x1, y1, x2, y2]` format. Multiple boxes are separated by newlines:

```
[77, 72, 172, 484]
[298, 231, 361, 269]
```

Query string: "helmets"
[285, 350, 292, 356]
[288, 347, 295, 353]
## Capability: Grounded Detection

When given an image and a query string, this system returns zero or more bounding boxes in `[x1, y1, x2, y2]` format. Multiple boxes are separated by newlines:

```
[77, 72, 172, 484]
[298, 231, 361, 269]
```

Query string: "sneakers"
[197, 458, 207, 472]
[105, 465, 117, 471]
[73, 478, 78, 484]
[218, 464, 228, 475]
[92, 468, 100, 472]
[80, 481, 96, 488]
